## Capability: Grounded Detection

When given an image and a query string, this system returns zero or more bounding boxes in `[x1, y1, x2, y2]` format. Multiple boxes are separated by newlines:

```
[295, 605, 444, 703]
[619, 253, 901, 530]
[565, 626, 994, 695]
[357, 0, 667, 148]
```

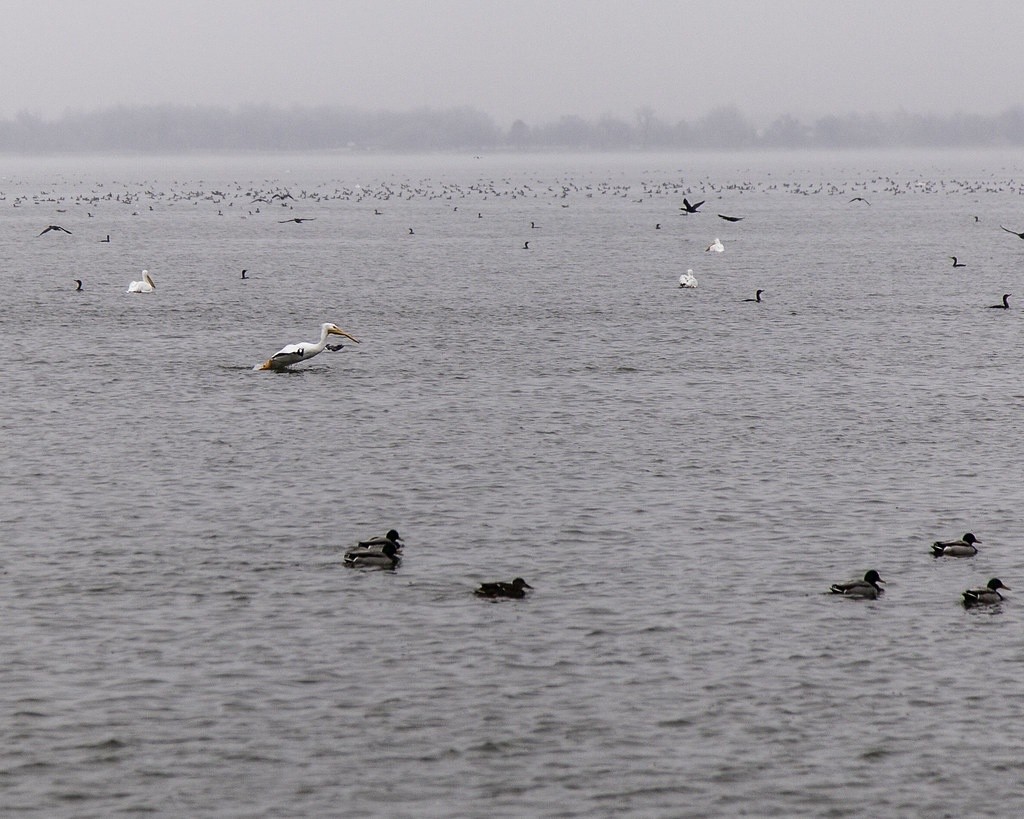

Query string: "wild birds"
[127, 269, 156, 294]
[656, 198, 705, 230]
[678, 269, 698, 288]
[931, 533, 982, 554]
[840, 165, 1024, 207]
[343, 530, 405, 566]
[0, 179, 209, 217]
[36, 225, 110, 242]
[74, 279, 83, 291]
[259, 322, 359, 371]
[705, 238, 724, 252]
[742, 289, 765, 303]
[561, 165, 838, 208]
[829, 570, 886, 595]
[474, 578, 535, 598]
[210, 171, 559, 249]
[961, 578, 1011, 603]
[949, 216, 1024, 308]
[718, 214, 744, 222]
[241, 270, 249, 279]
